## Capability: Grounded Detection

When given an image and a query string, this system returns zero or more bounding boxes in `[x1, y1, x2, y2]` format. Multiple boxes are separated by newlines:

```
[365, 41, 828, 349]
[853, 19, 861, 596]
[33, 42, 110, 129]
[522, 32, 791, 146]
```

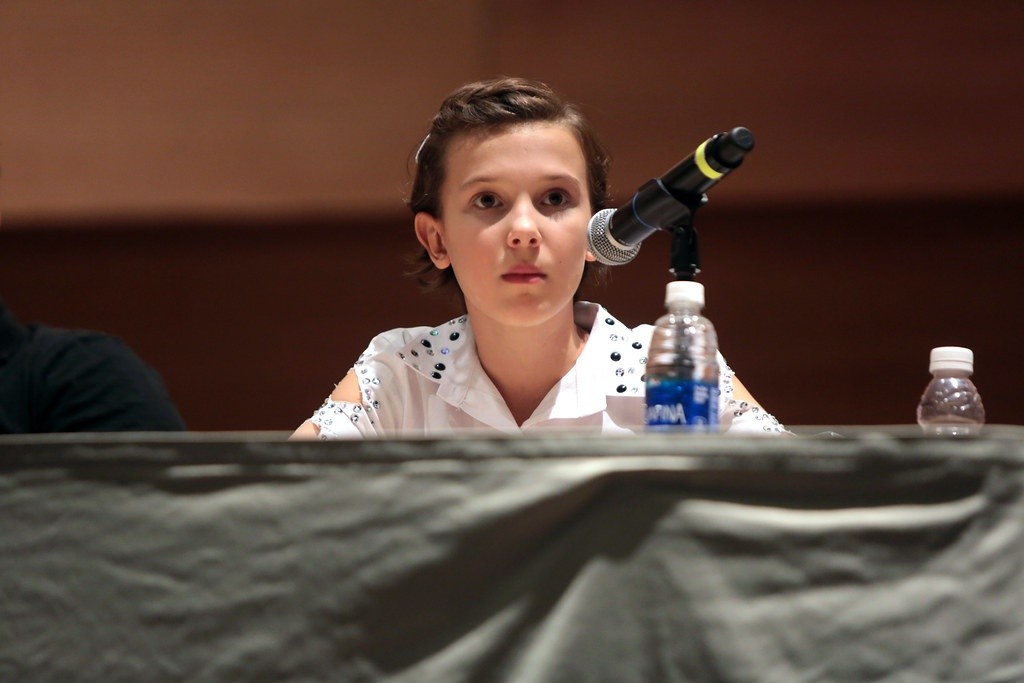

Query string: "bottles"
[644, 279, 719, 435]
[916, 345, 985, 438]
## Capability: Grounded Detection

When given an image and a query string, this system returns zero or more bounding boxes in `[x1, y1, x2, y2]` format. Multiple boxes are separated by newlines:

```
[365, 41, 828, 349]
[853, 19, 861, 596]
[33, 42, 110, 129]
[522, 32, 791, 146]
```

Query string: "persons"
[0, 303, 181, 430]
[288, 76, 798, 440]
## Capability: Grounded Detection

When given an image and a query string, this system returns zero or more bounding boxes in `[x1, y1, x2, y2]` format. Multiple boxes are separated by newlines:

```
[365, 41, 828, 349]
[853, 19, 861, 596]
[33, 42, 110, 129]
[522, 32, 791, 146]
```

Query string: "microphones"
[586, 128, 755, 266]
